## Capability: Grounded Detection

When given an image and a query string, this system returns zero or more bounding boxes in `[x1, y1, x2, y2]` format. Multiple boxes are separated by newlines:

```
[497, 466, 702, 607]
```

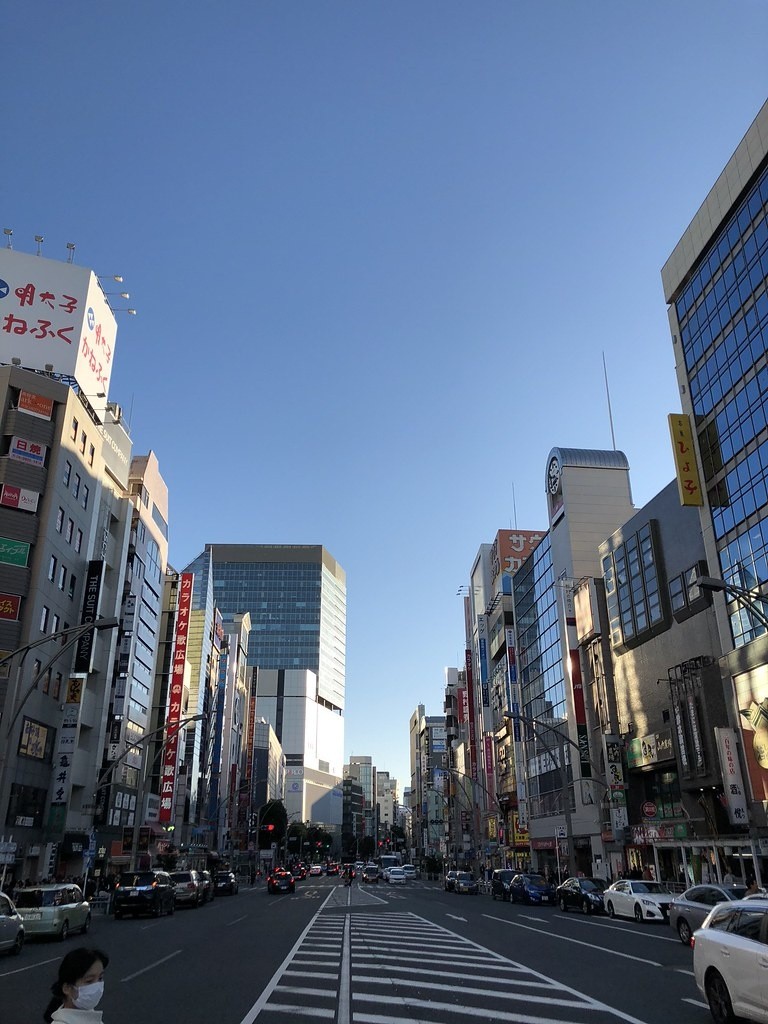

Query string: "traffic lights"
[262, 825, 274, 830]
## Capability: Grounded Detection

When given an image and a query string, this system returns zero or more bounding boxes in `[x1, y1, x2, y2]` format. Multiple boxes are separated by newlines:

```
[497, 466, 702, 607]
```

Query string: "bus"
[377, 855, 399, 873]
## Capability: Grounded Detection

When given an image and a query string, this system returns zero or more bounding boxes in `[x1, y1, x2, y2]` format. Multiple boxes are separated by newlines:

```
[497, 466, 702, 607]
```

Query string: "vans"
[490, 870, 521, 903]
[113, 872, 177, 920]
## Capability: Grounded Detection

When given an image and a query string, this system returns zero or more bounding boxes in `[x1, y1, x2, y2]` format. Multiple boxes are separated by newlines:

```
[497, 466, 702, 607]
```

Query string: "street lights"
[435, 763, 507, 848]
[128, 714, 208, 871]
[500, 710, 578, 877]
[256, 798, 286, 870]
[0, 616, 120, 784]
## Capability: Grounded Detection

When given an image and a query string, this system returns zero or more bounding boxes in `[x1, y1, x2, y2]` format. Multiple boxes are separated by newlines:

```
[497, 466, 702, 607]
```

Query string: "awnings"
[146, 821, 168, 836]
[210, 850, 220, 860]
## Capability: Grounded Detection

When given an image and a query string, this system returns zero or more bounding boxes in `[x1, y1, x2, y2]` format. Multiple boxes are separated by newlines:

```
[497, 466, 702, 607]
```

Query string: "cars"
[556, 876, 608, 915]
[168, 870, 240, 909]
[669, 884, 750, 947]
[509, 874, 557, 906]
[269, 861, 418, 893]
[603, 879, 683, 925]
[689, 901, 768, 1024]
[0, 891, 25, 955]
[444, 871, 479, 895]
[13, 884, 93, 942]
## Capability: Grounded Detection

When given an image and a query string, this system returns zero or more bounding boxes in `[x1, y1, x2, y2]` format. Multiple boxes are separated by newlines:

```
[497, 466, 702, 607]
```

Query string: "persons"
[679, 868, 691, 892]
[524, 867, 555, 889]
[618, 866, 653, 880]
[42, 946, 110, 1024]
[744, 876, 762, 897]
[724, 866, 736, 883]
[2, 871, 122, 901]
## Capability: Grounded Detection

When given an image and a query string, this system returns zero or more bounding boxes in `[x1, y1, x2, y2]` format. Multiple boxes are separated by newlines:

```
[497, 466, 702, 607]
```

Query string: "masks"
[67, 981, 104, 1009]
